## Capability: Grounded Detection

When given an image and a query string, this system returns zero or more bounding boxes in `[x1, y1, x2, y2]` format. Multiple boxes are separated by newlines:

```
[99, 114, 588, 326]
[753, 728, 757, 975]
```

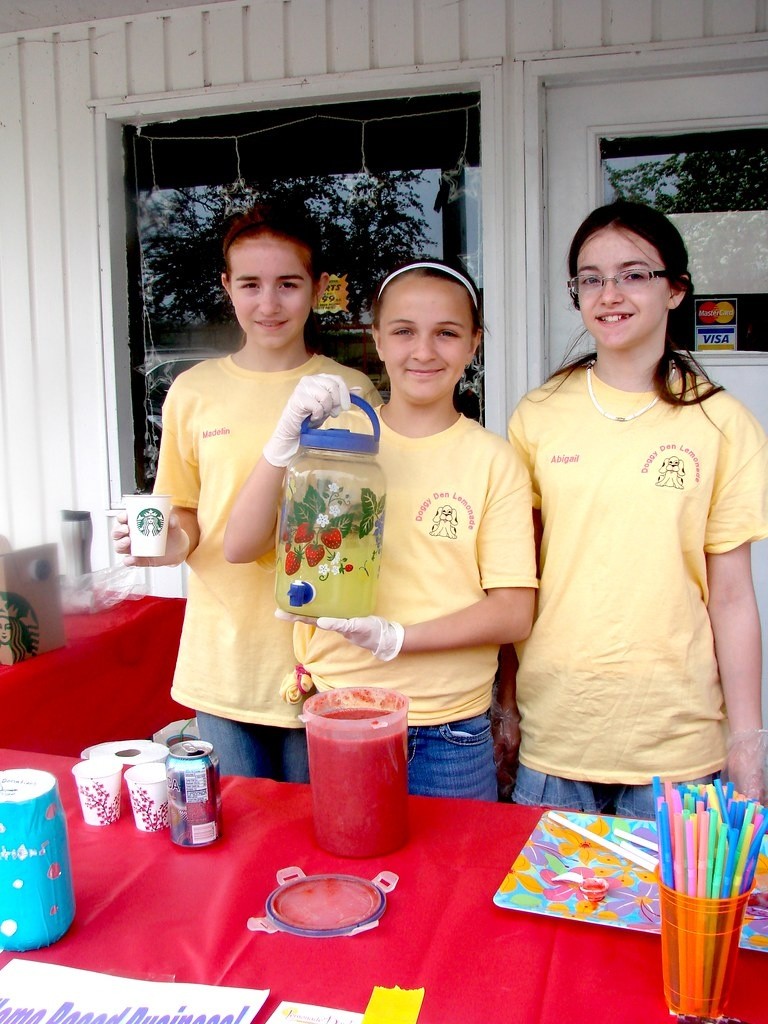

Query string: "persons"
[493, 201, 768, 819]
[114, 205, 384, 784]
[222, 260, 540, 806]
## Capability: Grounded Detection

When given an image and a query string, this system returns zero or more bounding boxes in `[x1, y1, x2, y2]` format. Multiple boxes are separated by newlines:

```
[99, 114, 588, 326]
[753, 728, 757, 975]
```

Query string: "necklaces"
[587, 358, 676, 422]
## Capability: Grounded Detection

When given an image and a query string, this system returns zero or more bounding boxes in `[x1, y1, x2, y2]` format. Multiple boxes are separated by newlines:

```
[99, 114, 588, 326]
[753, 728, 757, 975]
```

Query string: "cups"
[654, 862, 757, 1019]
[72, 757, 124, 825]
[124, 494, 172, 557]
[124, 762, 169, 832]
[297, 687, 411, 858]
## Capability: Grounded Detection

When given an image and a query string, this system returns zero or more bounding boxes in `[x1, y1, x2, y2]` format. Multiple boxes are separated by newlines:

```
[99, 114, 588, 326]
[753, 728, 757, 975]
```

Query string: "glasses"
[567, 268, 676, 297]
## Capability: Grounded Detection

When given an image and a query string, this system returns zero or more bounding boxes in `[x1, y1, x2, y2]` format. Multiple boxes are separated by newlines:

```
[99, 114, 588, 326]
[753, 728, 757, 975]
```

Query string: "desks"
[0, 594, 768, 1024]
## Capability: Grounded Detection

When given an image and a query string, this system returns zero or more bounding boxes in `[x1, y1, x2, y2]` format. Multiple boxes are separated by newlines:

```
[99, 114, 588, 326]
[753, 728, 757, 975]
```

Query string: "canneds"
[165, 740, 220, 848]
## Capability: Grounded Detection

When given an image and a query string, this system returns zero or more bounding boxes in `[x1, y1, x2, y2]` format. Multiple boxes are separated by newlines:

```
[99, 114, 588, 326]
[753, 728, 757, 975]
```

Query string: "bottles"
[275, 392, 388, 617]
[61, 510, 93, 589]
